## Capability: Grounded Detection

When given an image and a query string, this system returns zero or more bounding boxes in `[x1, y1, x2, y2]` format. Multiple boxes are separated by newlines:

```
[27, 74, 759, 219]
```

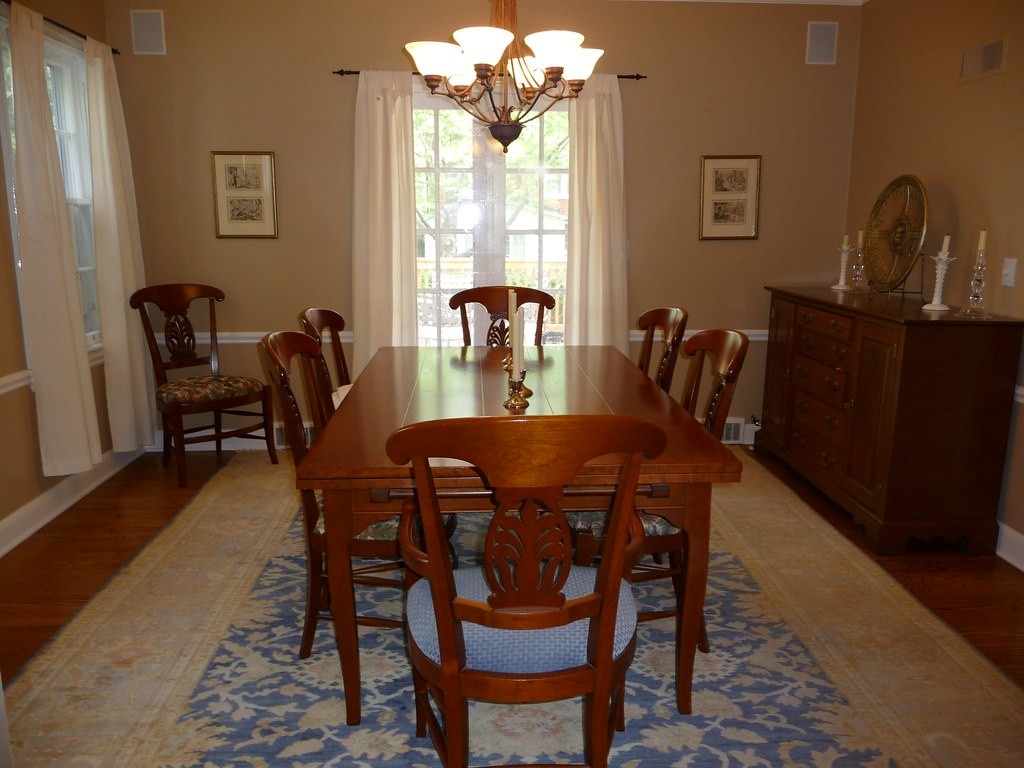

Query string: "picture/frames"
[699, 155, 761, 240]
[210, 150, 278, 239]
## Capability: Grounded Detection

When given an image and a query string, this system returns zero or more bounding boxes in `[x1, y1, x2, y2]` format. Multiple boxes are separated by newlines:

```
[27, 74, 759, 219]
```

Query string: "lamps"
[403, 0, 605, 154]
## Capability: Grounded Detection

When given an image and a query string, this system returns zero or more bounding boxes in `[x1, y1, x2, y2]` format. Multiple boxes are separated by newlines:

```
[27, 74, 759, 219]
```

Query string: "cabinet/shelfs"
[752, 285, 1024, 556]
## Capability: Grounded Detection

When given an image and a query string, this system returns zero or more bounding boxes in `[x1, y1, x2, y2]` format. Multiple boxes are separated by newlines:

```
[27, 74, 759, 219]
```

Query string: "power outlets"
[1001, 258, 1017, 288]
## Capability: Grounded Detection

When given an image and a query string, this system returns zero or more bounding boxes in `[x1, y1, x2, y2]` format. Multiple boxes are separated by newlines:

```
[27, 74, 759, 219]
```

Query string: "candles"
[978, 229, 988, 250]
[511, 292, 517, 313]
[508, 288, 515, 345]
[512, 313, 520, 379]
[858, 229, 863, 243]
[940, 232, 951, 254]
[518, 307, 525, 371]
[843, 232, 849, 248]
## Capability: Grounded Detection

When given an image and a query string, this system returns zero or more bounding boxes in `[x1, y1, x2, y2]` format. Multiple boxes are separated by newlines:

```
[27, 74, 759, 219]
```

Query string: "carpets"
[0, 450, 1024, 768]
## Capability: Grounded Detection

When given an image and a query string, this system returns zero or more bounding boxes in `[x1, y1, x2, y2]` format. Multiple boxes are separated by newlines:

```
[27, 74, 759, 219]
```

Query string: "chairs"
[576, 329, 749, 654]
[298, 308, 355, 416]
[448, 285, 557, 347]
[257, 331, 458, 660]
[129, 283, 280, 486]
[563, 307, 688, 565]
[386, 414, 668, 768]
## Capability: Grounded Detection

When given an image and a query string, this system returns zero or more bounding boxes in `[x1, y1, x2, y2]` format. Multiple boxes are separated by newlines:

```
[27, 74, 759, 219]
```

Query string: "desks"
[294, 346, 742, 727]
